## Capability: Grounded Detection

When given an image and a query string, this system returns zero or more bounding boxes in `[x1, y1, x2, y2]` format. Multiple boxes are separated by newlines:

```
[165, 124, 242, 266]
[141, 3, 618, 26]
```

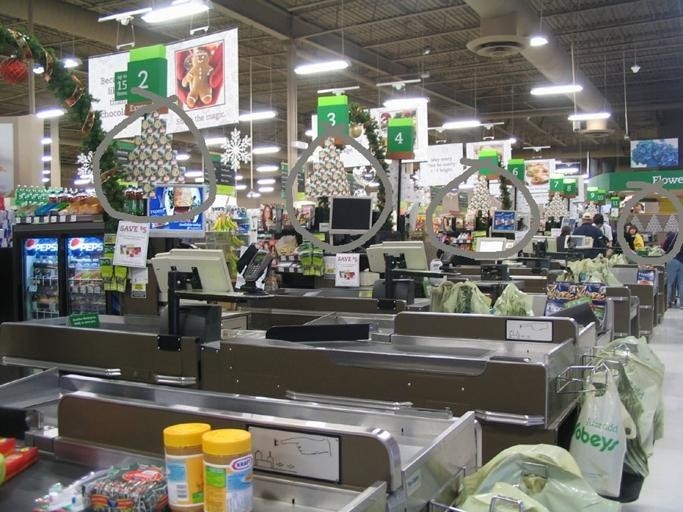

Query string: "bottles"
[13, 184, 146, 216]
[32, 253, 106, 313]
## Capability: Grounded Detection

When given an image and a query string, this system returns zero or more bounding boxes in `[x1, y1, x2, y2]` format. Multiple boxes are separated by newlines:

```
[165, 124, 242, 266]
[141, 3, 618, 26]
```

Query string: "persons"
[557, 212, 683, 310]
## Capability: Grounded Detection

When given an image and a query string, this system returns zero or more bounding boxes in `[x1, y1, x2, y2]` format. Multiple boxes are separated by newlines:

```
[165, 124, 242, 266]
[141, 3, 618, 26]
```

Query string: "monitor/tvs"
[475, 237, 506, 260]
[329, 196, 374, 235]
[151, 249, 234, 292]
[628, 136, 680, 170]
[564, 235, 586, 249]
[492, 210, 517, 233]
[147, 184, 205, 238]
[523, 236, 557, 254]
[366, 241, 429, 272]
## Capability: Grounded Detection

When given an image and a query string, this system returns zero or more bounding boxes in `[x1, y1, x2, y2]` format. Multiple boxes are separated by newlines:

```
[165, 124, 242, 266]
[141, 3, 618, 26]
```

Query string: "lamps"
[566, 32, 610, 123]
[428, 1, 581, 174]
[289, 1, 431, 161]
[34, 1, 284, 199]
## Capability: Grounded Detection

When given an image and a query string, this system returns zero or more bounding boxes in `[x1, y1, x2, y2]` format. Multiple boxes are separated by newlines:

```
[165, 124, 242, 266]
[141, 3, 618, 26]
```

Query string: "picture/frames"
[0, 116, 19, 197]
